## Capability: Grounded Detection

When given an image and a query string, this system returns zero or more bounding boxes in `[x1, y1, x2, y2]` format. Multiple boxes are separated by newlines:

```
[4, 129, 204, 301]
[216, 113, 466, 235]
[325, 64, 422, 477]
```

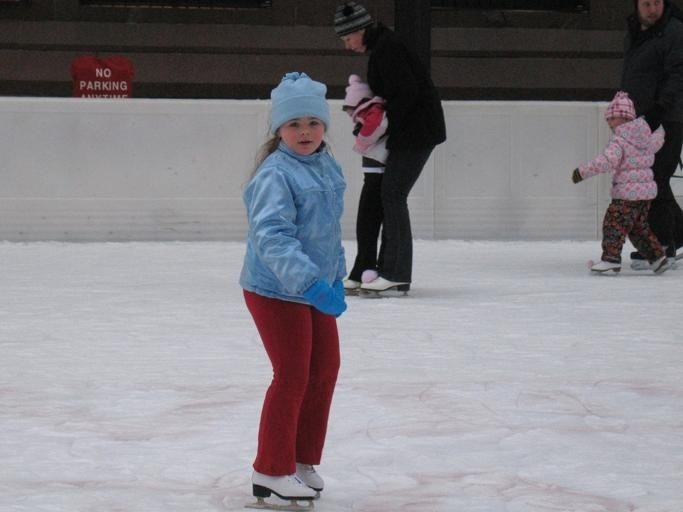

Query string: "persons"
[332, 0, 448, 293]
[571, 90, 671, 274]
[341, 73, 395, 291]
[618, 0, 683, 264]
[237, 69, 351, 502]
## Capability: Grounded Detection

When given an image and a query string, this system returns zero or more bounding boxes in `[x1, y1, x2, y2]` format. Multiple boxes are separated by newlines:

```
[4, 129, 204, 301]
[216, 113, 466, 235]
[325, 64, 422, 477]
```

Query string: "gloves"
[351, 123, 362, 135]
[333, 279, 344, 317]
[645, 104, 662, 133]
[303, 280, 346, 315]
[571, 168, 583, 183]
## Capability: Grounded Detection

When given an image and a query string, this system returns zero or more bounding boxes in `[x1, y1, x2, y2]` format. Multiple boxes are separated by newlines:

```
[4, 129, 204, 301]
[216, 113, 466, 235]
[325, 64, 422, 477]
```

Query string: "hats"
[269, 71, 330, 134]
[604, 91, 636, 120]
[343, 73, 373, 107]
[333, 1, 373, 36]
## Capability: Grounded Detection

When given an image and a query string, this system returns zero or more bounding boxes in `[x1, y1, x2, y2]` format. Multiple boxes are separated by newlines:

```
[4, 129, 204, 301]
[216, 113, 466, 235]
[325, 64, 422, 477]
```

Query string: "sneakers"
[253, 469, 316, 499]
[361, 276, 411, 291]
[296, 463, 324, 491]
[342, 279, 360, 288]
[591, 246, 682, 271]
